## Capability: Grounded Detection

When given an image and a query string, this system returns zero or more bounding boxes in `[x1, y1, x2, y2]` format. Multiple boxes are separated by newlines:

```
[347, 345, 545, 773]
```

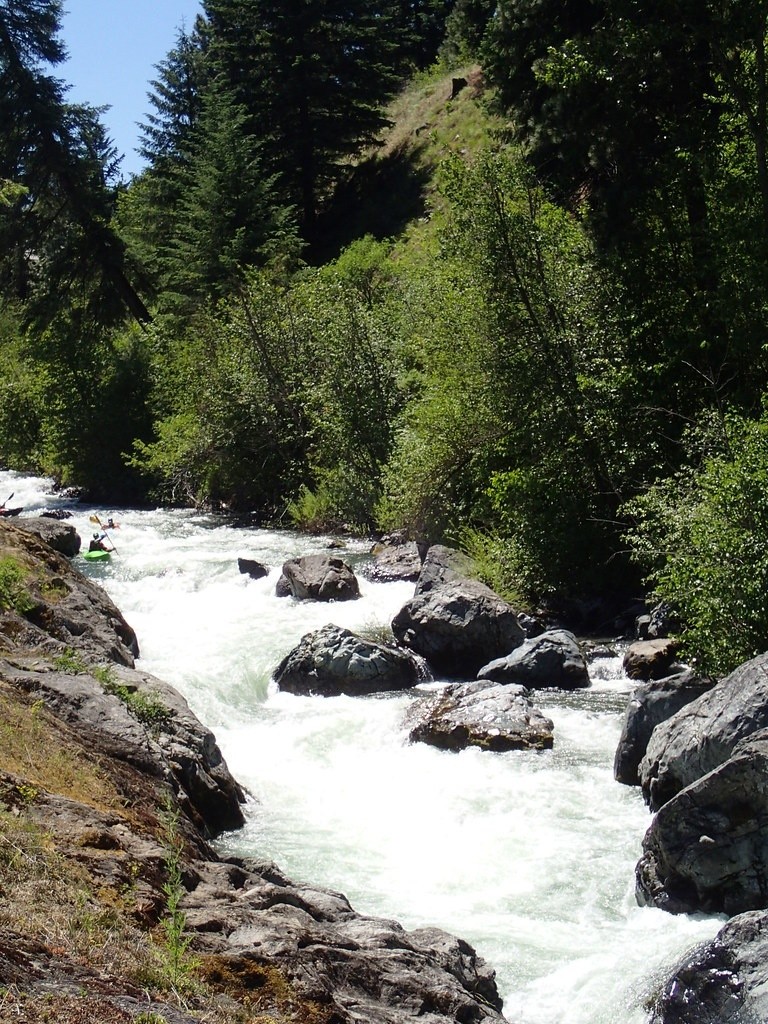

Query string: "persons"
[102, 518, 119, 529]
[89, 533, 116, 552]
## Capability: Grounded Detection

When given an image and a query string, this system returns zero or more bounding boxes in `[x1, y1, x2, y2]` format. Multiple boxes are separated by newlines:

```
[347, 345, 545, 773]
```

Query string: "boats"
[83, 550, 111, 561]
[0, 507, 24, 516]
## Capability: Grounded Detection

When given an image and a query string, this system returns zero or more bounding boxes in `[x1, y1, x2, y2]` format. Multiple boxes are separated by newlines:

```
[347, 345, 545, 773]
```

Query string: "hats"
[93, 533, 99, 538]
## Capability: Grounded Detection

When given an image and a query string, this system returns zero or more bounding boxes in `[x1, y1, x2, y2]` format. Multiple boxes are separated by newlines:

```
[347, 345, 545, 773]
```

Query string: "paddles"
[93, 513, 120, 555]
[89, 516, 108, 527]
